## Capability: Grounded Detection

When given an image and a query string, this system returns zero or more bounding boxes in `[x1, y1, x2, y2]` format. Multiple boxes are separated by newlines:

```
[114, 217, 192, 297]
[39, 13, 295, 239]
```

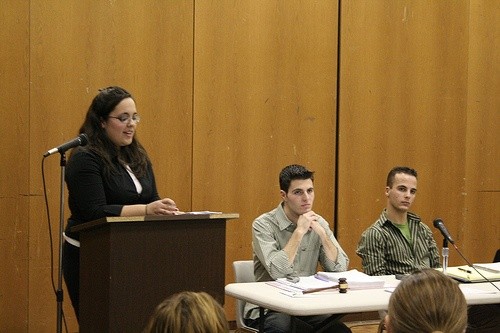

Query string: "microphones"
[42, 133, 89, 157]
[433, 217, 455, 244]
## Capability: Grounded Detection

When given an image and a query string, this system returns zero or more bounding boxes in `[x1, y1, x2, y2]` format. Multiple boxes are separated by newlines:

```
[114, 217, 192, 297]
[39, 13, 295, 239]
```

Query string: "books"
[265, 269, 384, 293]
[433, 264, 500, 284]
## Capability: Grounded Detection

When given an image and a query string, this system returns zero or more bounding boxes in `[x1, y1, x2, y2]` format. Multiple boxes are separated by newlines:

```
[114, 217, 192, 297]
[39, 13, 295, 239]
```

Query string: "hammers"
[301, 276, 349, 295]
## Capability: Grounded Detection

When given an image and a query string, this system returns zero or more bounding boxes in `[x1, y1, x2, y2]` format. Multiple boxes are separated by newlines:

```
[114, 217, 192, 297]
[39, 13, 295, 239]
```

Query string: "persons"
[355, 167, 440, 276]
[63, 86, 178, 333]
[252, 164, 352, 333]
[146, 292, 228, 333]
[378, 269, 468, 333]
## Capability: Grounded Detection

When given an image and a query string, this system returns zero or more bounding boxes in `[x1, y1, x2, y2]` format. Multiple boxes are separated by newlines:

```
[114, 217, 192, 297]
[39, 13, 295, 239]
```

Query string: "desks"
[225, 275, 500, 333]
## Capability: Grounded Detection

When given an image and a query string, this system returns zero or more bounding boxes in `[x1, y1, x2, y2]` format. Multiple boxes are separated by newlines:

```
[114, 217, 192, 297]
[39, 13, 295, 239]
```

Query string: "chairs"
[233, 260, 269, 333]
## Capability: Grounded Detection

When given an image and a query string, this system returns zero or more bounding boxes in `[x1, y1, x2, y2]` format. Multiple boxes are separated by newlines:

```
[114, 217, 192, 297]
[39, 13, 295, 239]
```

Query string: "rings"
[169, 205, 171, 210]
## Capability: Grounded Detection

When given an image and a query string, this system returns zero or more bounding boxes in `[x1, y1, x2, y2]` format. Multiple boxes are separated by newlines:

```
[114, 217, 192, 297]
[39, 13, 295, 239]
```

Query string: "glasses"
[108, 113, 140, 122]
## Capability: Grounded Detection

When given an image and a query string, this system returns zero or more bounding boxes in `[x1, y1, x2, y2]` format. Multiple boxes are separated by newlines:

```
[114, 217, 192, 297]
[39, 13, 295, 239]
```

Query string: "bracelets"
[145, 204, 148, 217]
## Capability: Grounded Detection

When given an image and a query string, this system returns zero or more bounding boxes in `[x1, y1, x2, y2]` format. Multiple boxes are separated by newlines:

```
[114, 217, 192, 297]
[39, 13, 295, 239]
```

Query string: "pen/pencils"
[457, 267, 472, 274]
